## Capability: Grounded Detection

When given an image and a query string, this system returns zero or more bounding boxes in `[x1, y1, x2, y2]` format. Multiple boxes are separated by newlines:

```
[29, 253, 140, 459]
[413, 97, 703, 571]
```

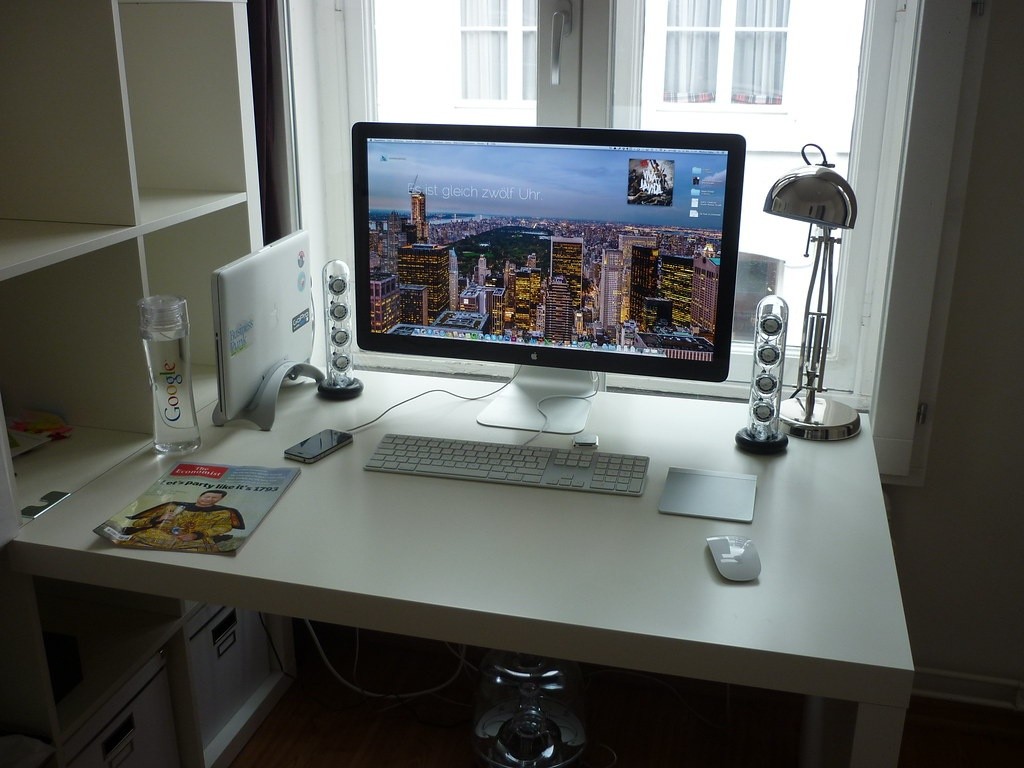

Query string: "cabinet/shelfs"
[0, 0, 294, 768]
[57, 652, 182, 768]
[163, 600, 271, 750]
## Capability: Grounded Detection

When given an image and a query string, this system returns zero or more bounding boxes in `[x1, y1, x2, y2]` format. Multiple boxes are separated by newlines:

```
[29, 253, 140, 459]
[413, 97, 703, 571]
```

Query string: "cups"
[140, 295, 202, 455]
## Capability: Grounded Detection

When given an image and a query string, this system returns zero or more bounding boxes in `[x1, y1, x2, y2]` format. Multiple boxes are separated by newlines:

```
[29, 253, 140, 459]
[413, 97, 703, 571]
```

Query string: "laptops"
[212, 230, 325, 430]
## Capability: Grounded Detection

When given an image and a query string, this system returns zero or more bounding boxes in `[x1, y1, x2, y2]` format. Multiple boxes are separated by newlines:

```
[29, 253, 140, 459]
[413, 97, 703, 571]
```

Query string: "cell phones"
[284, 429, 353, 464]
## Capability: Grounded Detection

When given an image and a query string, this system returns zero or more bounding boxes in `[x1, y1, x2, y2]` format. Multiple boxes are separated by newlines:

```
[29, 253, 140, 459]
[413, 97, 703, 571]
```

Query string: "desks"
[14, 369, 915, 768]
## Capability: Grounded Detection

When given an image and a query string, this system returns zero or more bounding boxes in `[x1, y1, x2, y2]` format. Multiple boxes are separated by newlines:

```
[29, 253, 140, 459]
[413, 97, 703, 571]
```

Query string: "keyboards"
[364, 434, 650, 496]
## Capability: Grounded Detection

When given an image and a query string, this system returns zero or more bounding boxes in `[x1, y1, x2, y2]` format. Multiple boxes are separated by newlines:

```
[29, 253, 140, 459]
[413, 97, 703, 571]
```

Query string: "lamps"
[763, 145, 863, 441]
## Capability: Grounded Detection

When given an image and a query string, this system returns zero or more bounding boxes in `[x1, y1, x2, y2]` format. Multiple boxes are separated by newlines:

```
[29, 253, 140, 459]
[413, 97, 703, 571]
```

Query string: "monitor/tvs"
[352, 121, 746, 432]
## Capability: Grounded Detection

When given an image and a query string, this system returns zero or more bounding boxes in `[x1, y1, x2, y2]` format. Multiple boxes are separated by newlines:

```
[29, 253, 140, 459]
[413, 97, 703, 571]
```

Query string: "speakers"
[317, 259, 363, 396]
[735, 295, 789, 453]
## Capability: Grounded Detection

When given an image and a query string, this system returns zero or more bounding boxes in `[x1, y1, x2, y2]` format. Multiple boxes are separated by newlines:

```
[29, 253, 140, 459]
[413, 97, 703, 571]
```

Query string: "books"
[93, 459, 301, 559]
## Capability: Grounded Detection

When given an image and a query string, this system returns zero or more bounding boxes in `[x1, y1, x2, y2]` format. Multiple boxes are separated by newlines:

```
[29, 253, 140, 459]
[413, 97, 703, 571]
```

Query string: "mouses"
[705, 536, 761, 581]
